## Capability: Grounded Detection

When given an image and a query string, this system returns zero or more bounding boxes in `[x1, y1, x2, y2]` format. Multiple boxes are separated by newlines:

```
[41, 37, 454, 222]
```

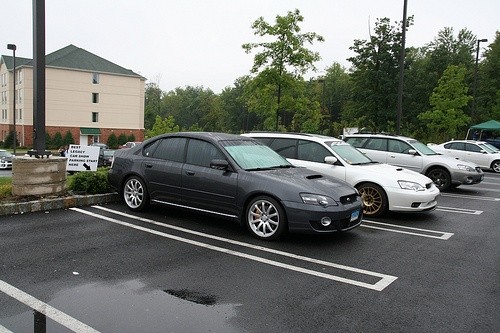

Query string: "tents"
[470, 120, 500, 142]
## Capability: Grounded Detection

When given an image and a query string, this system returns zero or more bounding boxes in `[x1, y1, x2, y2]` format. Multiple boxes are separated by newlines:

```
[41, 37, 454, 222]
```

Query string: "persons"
[58, 146, 69, 158]
[472, 130, 480, 141]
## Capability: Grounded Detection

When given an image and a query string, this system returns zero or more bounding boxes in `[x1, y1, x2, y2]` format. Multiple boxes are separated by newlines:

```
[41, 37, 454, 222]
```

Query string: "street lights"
[470, 38, 488, 126]
[7, 43, 17, 156]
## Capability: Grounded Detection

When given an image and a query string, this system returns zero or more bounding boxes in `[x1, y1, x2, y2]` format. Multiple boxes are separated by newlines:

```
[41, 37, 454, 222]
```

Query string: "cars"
[426, 140, 500, 174]
[0, 149, 17, 169]
[88, 143, 114, 166]
[108, 131, 365, 242]
[122, 141, 143, 149]
[334, 134, 486, 192]
[238, 133, 441, 218]
